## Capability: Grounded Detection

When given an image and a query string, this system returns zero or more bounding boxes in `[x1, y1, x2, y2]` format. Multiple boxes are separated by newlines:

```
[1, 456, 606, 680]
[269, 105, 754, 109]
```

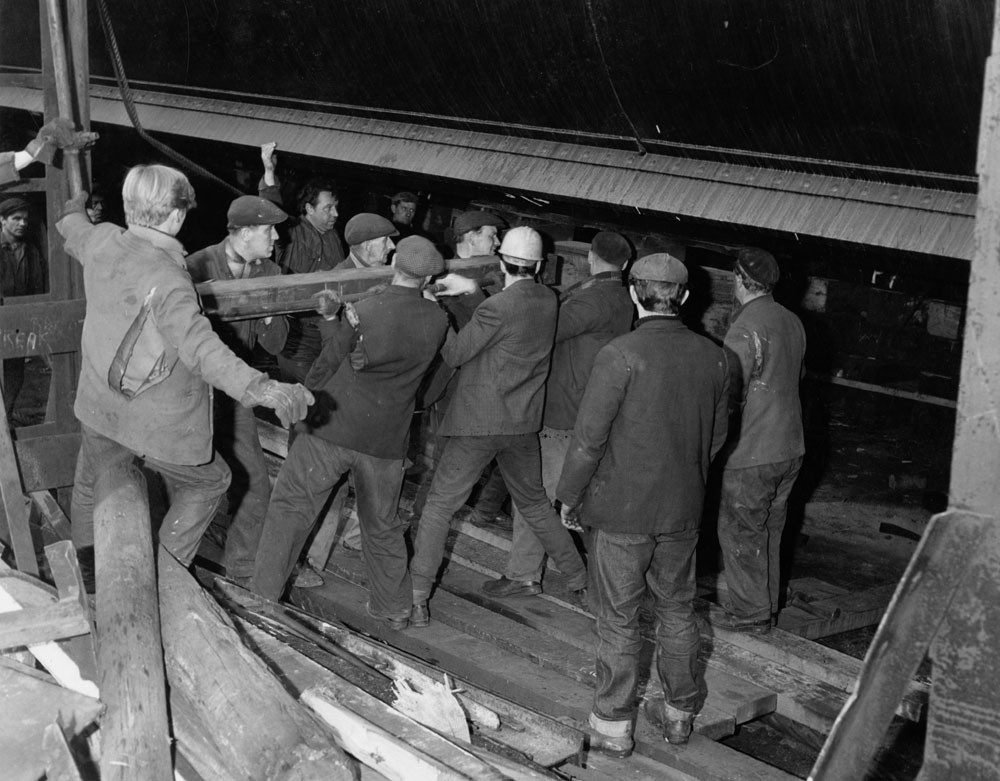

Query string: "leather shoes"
[704, 607, 776, 635]
[482, 575, 543, 597]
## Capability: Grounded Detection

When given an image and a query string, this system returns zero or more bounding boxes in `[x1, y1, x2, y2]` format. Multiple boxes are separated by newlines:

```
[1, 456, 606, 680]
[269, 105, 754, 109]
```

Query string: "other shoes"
[226, 572, 253, 593]
[343, 541, 363, 554]
[470, 508, 514, 532]
[555, 715, 634, 759]
[366, 599, 410, 631]
[573, 587, 591, 611]
[289, 560, 324, 588]
[407, 599, 429, 628]
[644, 697, 692, 746]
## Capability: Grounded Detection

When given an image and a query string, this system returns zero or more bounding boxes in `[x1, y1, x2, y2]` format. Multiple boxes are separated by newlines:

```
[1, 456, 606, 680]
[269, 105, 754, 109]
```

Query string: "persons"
[705, 249, 806, 638]
[0, 121, 632, 634]
[556, 253, 729, 759]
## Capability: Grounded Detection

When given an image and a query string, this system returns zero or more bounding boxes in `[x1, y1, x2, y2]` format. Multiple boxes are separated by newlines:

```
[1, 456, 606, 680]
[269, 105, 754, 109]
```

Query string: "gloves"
[25, 117, 76, 165]
[241, 373, 316, 430]
[310, 289, 342, 316]
[58, 191, 89, 220]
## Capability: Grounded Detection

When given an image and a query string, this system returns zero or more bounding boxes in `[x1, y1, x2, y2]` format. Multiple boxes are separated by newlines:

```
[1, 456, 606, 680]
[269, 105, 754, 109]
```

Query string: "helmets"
[498, 226, 544, 267]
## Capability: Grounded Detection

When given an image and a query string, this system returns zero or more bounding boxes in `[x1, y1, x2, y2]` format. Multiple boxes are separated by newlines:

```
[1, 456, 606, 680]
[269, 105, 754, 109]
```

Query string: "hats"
[344, 213, 401, 245]
[629, 253, 688, 285]
[227, 195, 288, 229]
[0, 196, 31, 217]
[454, 211, 510, 237]
[591, 231, 632, 266]
[396, 235, 445, 279]
[726, 247, 781, 290]
[392, 192, 419, 204]
